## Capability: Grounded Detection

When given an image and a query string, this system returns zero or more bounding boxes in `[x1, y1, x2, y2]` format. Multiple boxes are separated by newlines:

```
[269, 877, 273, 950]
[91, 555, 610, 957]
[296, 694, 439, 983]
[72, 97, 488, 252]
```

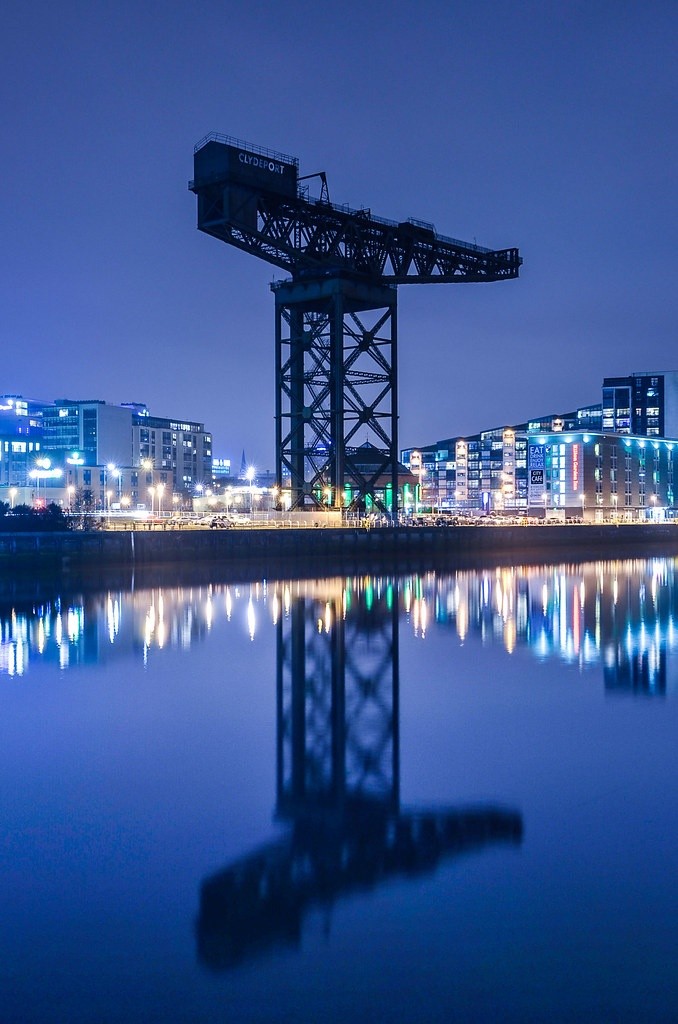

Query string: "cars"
[167, 512, 249, 529]
[407, 514, 584, 525]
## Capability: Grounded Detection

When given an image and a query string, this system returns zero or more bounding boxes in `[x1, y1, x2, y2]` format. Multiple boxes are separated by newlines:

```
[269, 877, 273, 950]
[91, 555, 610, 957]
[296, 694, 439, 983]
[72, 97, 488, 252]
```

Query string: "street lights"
[156, 484, 164, 520]
[9, 488, 18, 509]
[148, 486, 156, 515]
